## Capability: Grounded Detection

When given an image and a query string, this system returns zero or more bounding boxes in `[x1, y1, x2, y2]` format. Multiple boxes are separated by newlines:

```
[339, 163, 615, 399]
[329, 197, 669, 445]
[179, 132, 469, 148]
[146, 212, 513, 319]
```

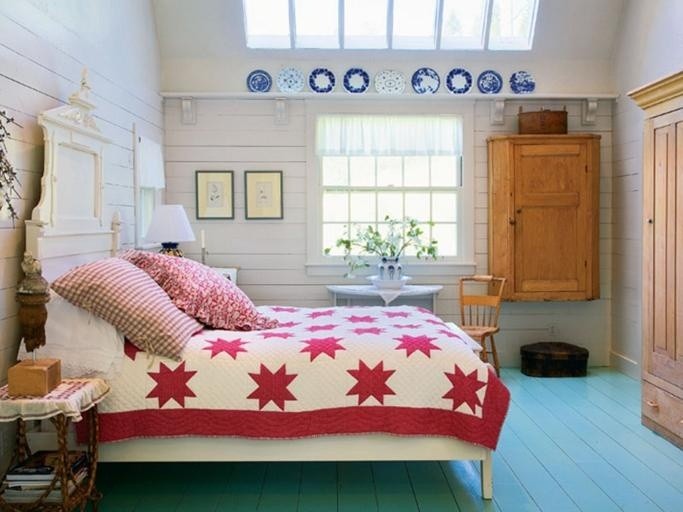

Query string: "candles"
[200, 228, 205, 264]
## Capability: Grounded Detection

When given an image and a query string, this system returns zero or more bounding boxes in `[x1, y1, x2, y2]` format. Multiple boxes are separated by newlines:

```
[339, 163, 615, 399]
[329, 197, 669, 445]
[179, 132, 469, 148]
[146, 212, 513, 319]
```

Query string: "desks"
[325, 285, 444, 316]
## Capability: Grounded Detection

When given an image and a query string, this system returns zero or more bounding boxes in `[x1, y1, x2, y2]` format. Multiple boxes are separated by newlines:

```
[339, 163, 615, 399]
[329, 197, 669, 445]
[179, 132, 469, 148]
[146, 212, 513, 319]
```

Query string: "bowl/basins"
[366, 276, 412, 290]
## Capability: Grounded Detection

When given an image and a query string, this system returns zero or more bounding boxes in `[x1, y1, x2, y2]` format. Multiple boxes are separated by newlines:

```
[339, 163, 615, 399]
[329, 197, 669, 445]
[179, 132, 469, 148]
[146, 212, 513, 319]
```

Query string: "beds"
[24, 66, 493, 500]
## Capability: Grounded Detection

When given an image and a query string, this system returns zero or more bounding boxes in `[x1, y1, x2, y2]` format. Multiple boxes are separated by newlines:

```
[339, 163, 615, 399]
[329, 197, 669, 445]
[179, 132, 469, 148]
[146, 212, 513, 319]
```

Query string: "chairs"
[459, 275, 507, 378]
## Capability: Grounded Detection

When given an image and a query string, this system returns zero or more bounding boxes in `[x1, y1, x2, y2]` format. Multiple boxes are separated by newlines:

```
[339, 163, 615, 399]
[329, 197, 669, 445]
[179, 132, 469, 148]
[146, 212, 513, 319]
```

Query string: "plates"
[410, 66, 441, 94]
[444, 66, 475, 96]
[508, 69, 537, 94]
[308, 67, 336, 94]
[341, 66, 372, 94]
[476, 68, 504, 94]
[246, 68, 273, 93]
[274, 65, 306, 94]
[373, 68, 406, 97]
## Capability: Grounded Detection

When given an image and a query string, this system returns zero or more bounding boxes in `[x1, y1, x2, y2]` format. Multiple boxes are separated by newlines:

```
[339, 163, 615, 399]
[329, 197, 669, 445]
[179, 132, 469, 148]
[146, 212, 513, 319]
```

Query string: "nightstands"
[208, 267, 237, 286]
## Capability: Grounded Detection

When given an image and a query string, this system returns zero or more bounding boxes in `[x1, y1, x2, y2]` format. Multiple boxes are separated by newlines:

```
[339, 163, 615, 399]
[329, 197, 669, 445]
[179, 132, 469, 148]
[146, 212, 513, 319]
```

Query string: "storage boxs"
[520, 342, 589, 377]
[518, 104, 568, 135]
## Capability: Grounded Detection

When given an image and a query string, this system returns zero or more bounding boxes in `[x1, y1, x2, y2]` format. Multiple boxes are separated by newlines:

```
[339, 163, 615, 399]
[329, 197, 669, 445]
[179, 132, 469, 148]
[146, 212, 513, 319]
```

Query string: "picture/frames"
[245, 171, 283, 219]
[196, 170, 235, 219]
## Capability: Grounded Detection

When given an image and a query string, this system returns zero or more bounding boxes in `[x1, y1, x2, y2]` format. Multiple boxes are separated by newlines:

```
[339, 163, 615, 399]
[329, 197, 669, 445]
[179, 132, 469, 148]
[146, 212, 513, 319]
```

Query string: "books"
[2, 451, 90, 503]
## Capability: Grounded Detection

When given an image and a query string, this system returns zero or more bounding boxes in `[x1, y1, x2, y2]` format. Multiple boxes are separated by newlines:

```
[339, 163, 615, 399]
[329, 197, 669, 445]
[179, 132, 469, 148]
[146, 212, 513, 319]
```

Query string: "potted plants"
[324, 215, 439, 280]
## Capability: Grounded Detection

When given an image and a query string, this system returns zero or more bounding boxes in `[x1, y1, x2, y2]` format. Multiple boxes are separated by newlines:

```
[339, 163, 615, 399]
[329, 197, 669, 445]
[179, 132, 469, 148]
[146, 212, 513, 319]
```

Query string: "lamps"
[143, 205, 196, 258]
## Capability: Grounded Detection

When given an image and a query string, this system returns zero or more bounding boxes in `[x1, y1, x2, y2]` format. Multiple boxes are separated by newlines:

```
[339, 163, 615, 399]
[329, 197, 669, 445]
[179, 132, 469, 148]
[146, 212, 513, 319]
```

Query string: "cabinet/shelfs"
[625, 70, 683, 450]
[0, 377, 101, 512]
[486, 134, 602, 301]
